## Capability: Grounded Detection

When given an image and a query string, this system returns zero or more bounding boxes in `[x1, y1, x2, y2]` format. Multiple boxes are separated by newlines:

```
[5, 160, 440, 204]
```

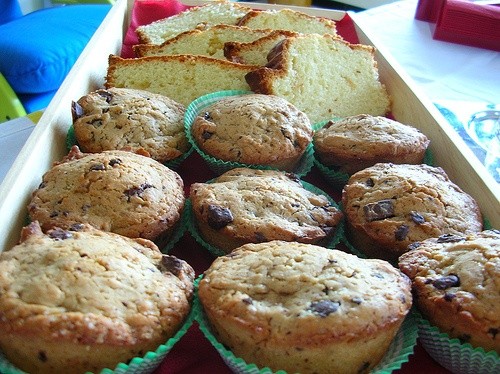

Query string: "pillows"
[0, 3, 114, 93]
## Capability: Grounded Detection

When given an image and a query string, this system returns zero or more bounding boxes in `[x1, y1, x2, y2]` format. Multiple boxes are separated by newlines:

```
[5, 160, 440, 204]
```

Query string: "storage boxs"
[0, 0, 500, 374]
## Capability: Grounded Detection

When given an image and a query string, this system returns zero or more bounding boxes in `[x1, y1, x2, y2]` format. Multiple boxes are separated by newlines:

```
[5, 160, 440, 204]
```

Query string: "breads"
[191, 167, 340, 253]
[313, 113, 430, 179]
[135, 0, 253, 44]
[191, 93, 314, 172]
[236, 8, 337, 37]
[197, 239, 413, 374]
[244, 36, 392, 126]
[224, 29, 299, 66]
[342, 161, 483, 263]
[106, 54, 266, 105]
[133, 24, 278, 60]
[27, 144, 185, 251]
[0, 221, 195, 374]
[397, 227, 500, 360]
[71, 87, 191, 161]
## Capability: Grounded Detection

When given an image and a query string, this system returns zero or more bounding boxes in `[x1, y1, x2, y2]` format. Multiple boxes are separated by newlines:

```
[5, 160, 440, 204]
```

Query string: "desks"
[0, 1, 500, 180]
